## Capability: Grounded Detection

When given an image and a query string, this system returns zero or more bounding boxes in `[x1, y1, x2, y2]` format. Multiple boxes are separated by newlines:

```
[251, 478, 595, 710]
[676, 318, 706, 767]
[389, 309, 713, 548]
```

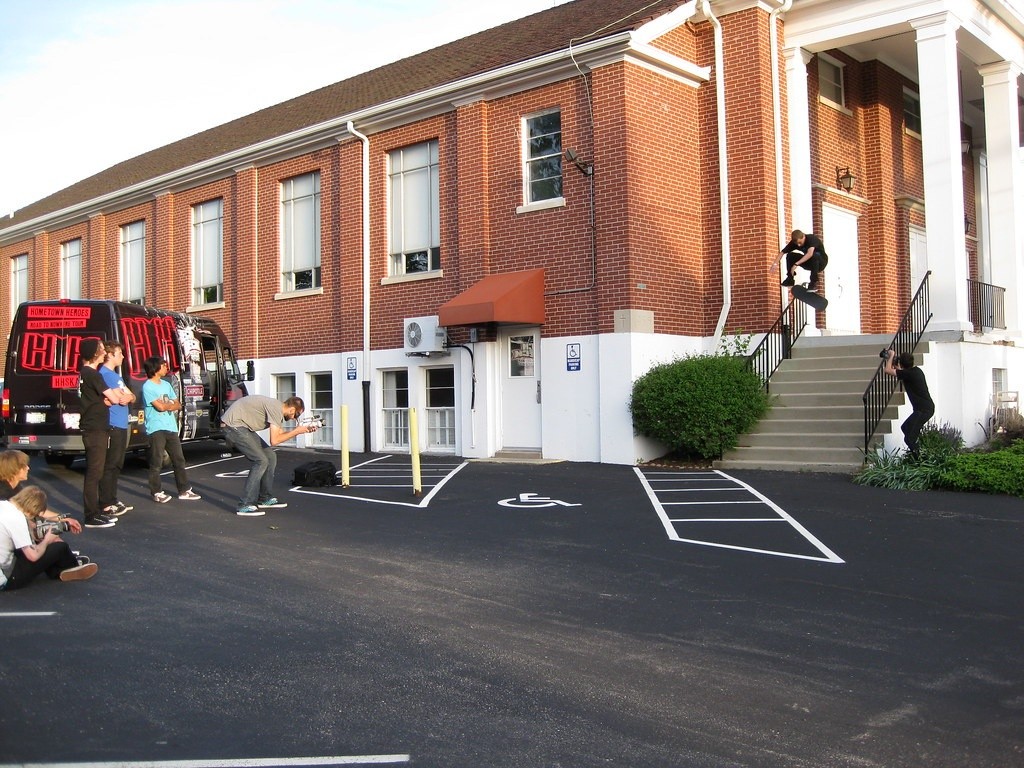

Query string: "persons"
[0, 486, 98, 591]
[0, 450, 82, 535]
[79, 338, 120, 528]
[98, 340, 136, 515]
[885, 350, 935, 452]
[770, 230, 828, 293]
[142, 357, 201, 503]
[220, 395, 318, 516]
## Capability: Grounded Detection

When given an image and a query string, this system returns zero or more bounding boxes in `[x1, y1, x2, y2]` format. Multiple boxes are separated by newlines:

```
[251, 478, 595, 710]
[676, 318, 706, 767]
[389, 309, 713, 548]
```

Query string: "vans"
[2, 299, 256, 465]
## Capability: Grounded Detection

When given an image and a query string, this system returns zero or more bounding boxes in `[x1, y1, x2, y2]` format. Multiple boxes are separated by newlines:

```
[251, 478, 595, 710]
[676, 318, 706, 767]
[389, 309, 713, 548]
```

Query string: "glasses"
[294, 410, 300, 419]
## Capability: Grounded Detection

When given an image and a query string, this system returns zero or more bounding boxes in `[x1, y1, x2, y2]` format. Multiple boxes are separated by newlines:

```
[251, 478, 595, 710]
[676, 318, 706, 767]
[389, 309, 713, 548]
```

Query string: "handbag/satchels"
[291, 461, 338, 487]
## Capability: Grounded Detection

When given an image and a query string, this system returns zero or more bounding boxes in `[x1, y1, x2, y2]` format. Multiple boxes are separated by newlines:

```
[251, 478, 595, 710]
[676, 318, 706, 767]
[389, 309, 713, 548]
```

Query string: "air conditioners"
[403, 315, 447, 352]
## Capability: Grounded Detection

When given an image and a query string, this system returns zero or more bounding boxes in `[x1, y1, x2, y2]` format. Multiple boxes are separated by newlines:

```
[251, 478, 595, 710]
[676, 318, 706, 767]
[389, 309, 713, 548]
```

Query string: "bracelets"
[794, 264, 798, 266]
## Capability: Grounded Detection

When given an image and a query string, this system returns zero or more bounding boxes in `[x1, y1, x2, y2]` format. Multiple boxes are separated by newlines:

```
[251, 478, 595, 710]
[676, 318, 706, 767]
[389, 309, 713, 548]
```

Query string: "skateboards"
[791, 284, 828, 312]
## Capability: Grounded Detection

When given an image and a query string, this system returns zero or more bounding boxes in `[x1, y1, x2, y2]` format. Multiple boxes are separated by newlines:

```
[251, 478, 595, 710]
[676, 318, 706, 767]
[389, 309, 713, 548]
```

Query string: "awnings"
[440, 269, 545, 327]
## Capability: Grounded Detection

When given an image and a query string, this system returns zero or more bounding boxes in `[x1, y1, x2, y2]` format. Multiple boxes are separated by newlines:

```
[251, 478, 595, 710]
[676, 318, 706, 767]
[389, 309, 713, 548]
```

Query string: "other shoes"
[75, 555, 91, 566]
[60, 563, 97, 581]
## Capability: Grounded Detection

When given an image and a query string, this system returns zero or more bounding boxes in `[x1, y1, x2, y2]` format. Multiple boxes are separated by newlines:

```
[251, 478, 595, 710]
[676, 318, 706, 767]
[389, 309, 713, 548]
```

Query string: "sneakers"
[152, 490, 172, 503]
[258, 497, 287, 508]
[101, 503, 127, 515]
[236, 504, 266, 516]
[99, 514, 118, 522]
[178, 487, 201, 500]
[84, 516, 116, 528]
[115, 499, 134, 510]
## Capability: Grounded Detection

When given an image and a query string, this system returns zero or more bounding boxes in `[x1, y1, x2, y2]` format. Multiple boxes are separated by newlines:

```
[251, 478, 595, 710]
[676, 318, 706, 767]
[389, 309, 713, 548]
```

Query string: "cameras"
[33, 512, 71, 539]
[296, 415, 326, 432]
[879, 348, 899, 368]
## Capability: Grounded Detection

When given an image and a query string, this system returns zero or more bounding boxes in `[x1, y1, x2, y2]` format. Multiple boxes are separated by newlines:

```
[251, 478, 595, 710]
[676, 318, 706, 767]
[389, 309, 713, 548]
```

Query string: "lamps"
[961, 137, 971, 155]
[964, 215, 972, 234]
[835, 165, 857, 195]
[563, 148, 593, 177]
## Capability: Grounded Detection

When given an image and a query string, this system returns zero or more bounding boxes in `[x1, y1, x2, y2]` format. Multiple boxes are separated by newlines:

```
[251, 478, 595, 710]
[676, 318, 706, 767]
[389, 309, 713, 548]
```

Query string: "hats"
[79, 338, 99, 361]
